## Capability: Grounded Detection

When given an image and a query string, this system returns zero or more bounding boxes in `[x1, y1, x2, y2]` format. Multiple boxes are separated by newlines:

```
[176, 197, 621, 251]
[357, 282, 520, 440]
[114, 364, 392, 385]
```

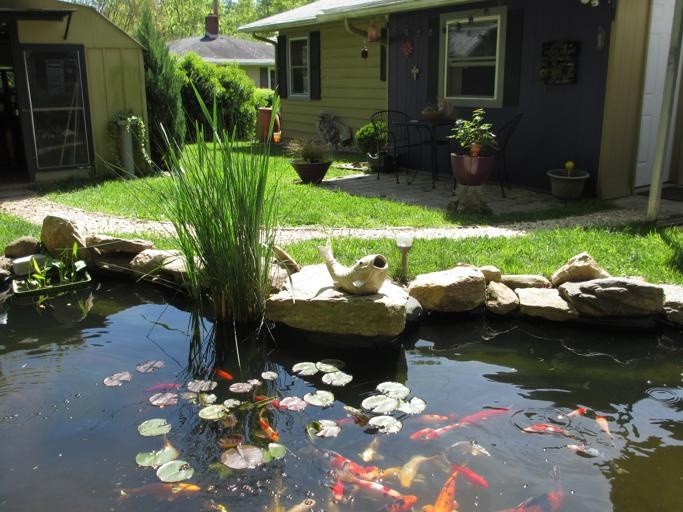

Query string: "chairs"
[370, 110, 423, 184]
[452, 112, 523, 198]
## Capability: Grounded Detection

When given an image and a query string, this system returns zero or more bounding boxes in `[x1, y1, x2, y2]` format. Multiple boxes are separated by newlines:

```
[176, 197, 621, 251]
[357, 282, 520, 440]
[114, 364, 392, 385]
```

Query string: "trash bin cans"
[256, 107, 272, 143]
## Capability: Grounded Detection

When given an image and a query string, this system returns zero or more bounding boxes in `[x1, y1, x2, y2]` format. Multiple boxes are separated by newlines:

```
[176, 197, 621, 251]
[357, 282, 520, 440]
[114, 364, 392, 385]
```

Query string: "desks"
[391, 120, 455, 188]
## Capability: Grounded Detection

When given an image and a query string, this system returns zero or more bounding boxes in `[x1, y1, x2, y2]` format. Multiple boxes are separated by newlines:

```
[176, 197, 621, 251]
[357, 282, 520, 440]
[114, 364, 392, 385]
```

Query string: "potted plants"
[450, 109, 501, 186]
[285, 137, 333, 184]
[547, 161, 590, 201]
